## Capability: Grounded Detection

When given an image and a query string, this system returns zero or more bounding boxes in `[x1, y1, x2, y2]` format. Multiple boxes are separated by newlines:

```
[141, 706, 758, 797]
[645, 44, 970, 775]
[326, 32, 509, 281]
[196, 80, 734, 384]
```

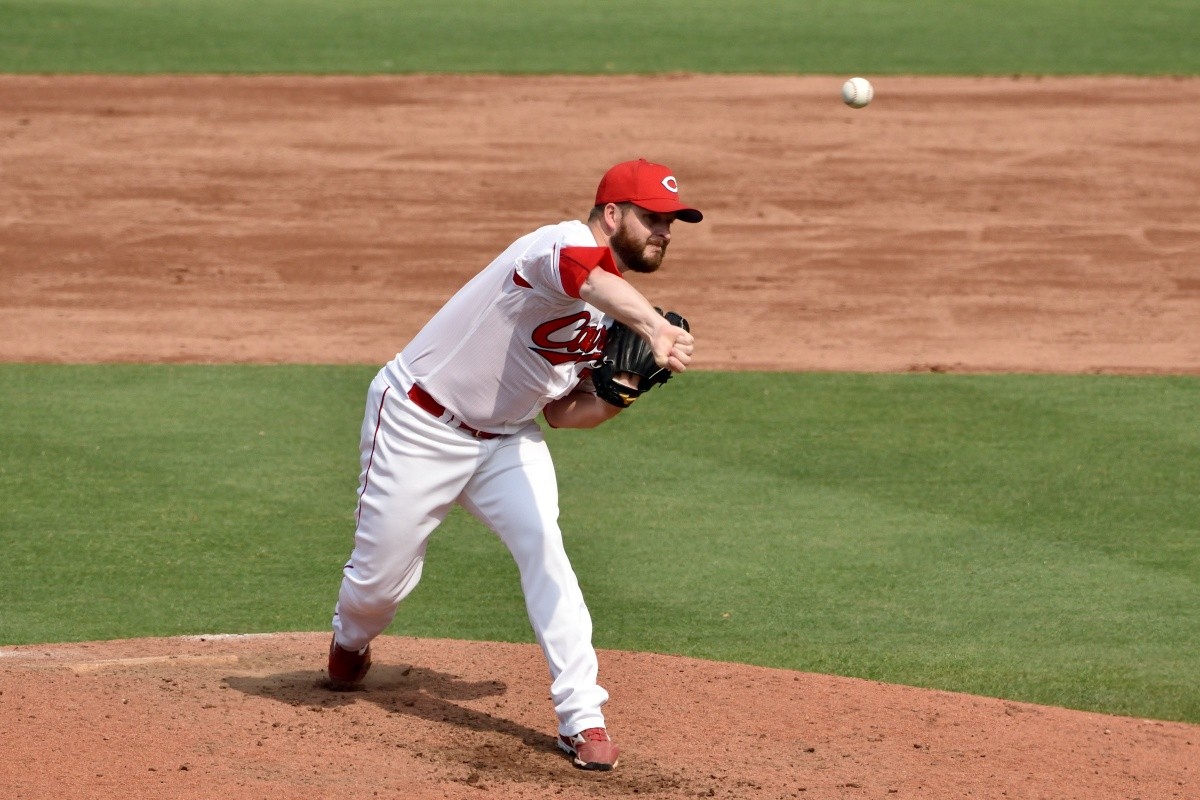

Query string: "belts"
[407, 382, 503, 441]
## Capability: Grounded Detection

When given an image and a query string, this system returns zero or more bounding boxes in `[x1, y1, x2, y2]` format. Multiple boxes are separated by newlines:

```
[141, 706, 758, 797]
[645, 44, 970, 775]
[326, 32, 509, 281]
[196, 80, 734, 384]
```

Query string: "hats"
[596, 158, 705, 223]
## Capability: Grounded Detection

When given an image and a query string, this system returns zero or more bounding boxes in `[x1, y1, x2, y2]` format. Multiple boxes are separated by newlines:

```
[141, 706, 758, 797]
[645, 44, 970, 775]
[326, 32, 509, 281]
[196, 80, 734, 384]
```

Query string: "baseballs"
[842, 78, 875, 108]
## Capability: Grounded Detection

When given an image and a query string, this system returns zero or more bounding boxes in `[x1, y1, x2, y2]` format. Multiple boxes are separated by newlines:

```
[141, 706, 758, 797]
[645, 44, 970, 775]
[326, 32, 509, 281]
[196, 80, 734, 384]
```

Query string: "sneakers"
[327, 630, 373, 687]
[556, 727, 621, 771]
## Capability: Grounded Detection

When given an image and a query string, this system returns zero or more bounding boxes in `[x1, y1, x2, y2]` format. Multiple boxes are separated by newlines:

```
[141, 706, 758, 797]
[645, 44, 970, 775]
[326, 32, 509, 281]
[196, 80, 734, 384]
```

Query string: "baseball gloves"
[592, 306, 690, 408]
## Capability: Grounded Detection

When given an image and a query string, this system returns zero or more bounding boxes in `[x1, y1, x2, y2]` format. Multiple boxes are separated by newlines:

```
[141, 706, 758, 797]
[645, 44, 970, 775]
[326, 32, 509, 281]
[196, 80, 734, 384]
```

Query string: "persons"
[328, 158, 703, 771]
